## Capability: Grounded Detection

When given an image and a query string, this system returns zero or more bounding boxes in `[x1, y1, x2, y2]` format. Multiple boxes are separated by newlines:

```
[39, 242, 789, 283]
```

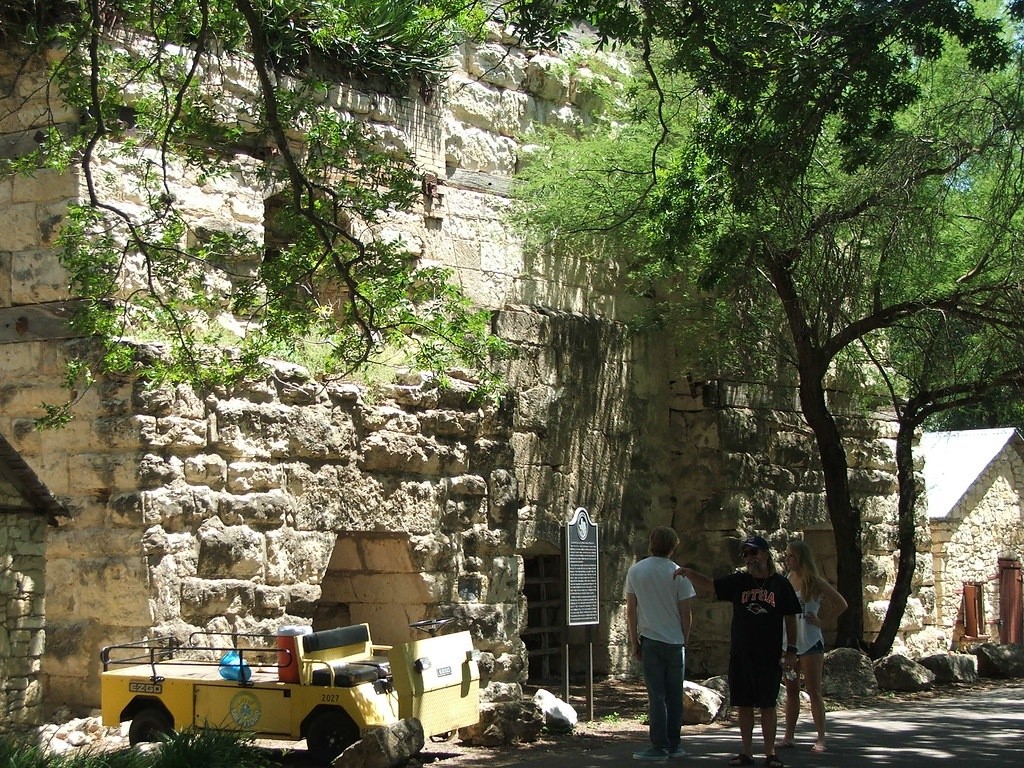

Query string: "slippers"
[765, 754, 783, 768]
[774, 740, 791, 749]
[728, 753, 755, 768]
[811, 743, 826, 753]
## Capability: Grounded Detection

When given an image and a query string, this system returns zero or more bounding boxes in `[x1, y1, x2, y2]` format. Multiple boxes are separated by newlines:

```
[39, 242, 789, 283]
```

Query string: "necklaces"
[753, 577, 767, 595]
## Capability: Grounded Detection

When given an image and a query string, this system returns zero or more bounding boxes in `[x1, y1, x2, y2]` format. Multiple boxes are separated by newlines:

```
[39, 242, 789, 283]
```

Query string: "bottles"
[779, 658, 797, 681]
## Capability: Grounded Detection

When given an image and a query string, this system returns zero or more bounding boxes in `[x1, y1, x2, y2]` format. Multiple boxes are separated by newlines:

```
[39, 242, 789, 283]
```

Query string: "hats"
[740, 536, 769, 550]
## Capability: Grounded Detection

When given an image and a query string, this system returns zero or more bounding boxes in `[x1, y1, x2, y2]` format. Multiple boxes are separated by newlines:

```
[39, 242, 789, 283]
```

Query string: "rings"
[788, 666, 790, 668]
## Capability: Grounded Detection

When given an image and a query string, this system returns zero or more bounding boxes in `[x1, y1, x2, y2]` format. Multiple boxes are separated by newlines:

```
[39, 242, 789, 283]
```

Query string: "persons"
[774, 540, 849, 751]
[626, 526, 697, 760]
[672, 536, 802, 768]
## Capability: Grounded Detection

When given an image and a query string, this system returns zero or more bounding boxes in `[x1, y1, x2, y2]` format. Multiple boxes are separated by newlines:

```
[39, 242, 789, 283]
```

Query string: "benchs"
[293, 622, 392, 688]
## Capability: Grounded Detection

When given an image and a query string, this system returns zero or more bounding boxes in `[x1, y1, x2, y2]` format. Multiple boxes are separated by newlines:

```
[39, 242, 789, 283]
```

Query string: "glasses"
[741, 548, 758, 558]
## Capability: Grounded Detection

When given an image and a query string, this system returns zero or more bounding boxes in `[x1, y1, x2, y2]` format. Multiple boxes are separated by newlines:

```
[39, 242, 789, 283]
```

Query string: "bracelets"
[787, 644, 798, 650]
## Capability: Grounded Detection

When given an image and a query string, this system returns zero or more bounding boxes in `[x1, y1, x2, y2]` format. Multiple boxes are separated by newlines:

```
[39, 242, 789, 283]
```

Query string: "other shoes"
[633, 745, 670, 760]
[669, 747, 685, 757]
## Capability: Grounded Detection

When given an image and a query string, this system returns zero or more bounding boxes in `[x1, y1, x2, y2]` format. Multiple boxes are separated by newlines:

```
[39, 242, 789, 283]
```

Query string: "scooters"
[99, 613, 487, 758]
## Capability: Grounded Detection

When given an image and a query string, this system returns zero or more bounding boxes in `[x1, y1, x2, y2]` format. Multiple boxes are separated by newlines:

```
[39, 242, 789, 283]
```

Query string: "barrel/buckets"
[276, 625, 313, 682]
[217, 650, 252, 682]
